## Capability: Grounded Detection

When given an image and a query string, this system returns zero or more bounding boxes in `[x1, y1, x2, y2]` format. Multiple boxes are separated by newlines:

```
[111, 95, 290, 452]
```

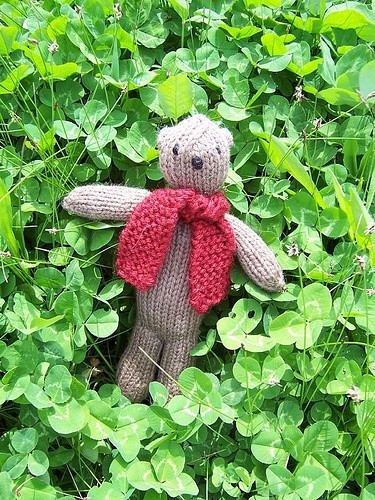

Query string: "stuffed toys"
[64, 114, 285, 405]
